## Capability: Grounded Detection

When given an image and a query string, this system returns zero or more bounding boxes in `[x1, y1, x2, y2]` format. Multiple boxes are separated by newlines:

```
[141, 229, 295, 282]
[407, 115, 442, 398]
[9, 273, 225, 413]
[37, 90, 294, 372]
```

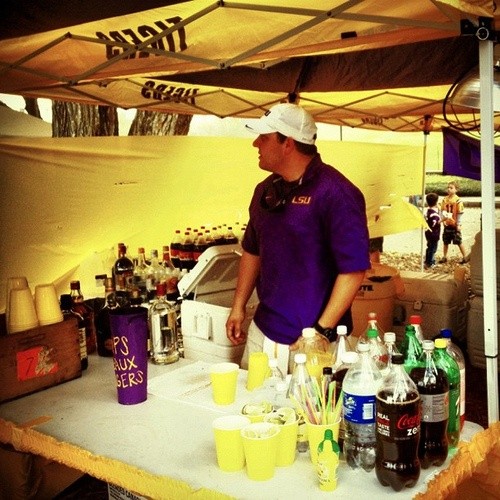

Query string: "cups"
[264, 410, 300, 467]
[108, 306, 149, 405]
[246, 352, 268, 391]
[209, 362, 239, 405]
[211, 415, 251, 472]
[239, 399, 275, 423]
[303, 412, 342, 466]
[6, 276, 64, 335]
[240, 422, 282, 481]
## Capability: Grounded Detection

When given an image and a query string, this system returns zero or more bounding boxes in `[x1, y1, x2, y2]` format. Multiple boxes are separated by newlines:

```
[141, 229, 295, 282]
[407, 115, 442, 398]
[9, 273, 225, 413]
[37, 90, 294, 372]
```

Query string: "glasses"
[259, 182, 292, 216]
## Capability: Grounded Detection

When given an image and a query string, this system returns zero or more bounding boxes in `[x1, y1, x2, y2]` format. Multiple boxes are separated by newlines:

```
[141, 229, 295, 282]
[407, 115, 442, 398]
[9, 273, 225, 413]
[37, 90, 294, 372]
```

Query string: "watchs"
[314, 320, 337, 342]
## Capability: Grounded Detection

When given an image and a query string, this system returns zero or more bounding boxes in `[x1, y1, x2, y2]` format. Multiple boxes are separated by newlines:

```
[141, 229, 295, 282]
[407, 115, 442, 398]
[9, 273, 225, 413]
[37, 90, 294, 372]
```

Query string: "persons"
[438, 181, 467, 264]
[425, 193, 448, 268]
[225, 102, 372, 374]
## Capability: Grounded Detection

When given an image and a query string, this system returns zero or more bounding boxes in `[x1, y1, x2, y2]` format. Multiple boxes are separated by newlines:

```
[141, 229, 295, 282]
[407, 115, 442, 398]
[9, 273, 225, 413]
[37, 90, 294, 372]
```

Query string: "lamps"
[448, 59, 500, 112]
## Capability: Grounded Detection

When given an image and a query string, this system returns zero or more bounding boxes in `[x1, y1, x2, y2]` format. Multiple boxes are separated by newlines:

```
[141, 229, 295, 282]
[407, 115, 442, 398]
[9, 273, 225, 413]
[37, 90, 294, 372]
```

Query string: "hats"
[245, 104, 318, 147]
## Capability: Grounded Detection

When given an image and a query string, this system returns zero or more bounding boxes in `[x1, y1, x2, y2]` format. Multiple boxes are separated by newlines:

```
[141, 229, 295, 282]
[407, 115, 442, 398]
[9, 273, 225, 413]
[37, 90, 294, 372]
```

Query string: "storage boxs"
[177, 244, 260, 365]
[0, 313, 82, 403]
[392, 229, 500, 369]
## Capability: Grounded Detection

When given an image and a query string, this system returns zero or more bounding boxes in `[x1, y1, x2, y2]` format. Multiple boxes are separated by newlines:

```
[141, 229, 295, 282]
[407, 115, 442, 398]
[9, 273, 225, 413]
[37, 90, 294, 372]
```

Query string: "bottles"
[261, 312, 466, 492]
[69, 281, 95, 355]
[95, 222, 248, 365]
[60, 294, 88, 370]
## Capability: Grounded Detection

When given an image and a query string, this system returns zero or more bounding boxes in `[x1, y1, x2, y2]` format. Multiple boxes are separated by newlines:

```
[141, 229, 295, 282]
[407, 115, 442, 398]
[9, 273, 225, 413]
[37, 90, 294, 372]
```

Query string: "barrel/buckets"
[470, 228, 500, 300]
[348, 262, 404, 351]
[467, 294, 500, 371]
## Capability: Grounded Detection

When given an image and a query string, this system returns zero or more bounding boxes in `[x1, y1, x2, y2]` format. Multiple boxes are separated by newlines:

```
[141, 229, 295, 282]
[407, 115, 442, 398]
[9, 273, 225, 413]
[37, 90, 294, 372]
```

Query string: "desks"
[0, 354, 486, 500]
